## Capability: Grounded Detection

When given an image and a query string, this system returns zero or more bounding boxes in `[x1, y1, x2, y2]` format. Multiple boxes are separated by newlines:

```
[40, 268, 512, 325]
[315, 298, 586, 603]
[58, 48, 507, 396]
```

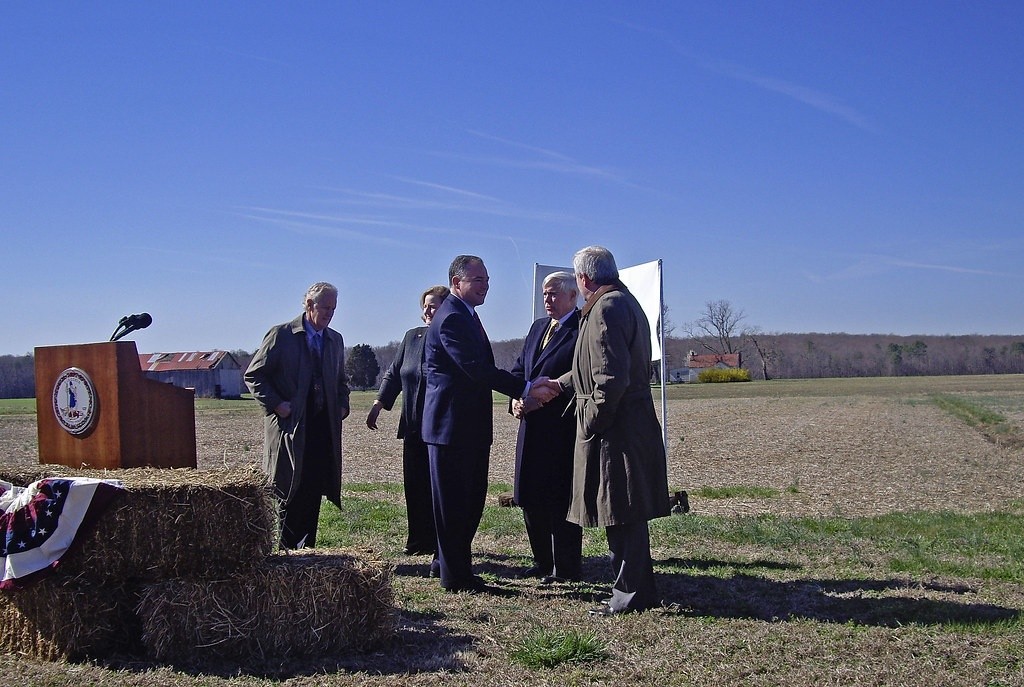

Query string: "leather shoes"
[590, 598, 617, 615]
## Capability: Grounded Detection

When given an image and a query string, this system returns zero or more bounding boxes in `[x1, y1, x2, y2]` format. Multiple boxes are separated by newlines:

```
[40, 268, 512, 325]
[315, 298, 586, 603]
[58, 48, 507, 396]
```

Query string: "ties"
[541, 321, 559, 350]
[313, 334, 321, 354]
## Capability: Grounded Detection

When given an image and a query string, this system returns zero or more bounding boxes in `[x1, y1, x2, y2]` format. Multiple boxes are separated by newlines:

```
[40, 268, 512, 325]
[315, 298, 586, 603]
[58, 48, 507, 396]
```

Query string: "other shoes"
[430, 571, 439, 578]
[523, 564, 553, 577]
[446, 583, 484, 593]
[403, 547, 435, 556]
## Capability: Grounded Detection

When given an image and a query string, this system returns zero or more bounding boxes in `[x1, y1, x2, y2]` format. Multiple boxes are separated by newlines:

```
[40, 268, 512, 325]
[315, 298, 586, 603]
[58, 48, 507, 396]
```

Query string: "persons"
[242, 281, 351, 550]
[533, 246, 671, 616]
[508, 271, 583, 581]
[366, 285, 451, 555]
[420, 255, 558, 593]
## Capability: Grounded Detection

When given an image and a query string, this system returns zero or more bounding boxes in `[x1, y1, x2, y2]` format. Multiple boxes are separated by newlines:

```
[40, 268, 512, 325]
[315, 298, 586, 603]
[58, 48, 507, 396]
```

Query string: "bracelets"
[374, 400, 383, 408]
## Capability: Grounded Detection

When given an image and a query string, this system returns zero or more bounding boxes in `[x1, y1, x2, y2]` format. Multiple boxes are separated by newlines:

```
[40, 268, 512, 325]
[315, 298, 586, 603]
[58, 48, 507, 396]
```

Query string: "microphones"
[121, 313, 152, 329]
